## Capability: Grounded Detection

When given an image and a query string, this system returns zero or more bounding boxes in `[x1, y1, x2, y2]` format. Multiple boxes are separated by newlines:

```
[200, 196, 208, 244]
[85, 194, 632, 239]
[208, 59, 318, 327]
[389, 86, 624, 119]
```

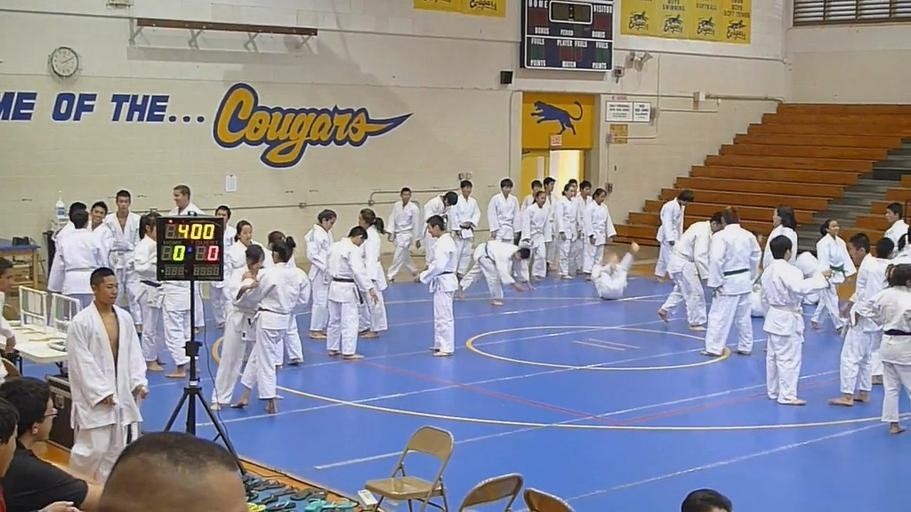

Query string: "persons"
[66, 267, 149, 485]
[654, 190, 694, 283]
[413, 215, 457, 356]
[458, 240, 535, 307]
[658, 202, 911, 436]
[0, 400, 82, 512]
[680, 488, 732, 512]
[48, 184, 313, 414]
[386, 180, 481, 283]
[0, 376, 105, 512]
[304, 207, 389, 360]
[590, 240, 640, 300]
[97, 433, 248, 512]
[487, 176, 618, 284]
[0, 256, 16, 385]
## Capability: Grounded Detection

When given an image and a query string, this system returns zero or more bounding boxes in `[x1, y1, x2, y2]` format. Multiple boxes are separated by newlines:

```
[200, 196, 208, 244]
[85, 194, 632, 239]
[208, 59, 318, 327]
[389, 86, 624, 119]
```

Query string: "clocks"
[51, 46, 79, 77]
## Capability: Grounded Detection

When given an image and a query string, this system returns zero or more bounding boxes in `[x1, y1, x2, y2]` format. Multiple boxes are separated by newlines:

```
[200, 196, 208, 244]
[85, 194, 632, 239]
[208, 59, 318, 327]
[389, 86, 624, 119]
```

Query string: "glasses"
[40, 408, 60, 419]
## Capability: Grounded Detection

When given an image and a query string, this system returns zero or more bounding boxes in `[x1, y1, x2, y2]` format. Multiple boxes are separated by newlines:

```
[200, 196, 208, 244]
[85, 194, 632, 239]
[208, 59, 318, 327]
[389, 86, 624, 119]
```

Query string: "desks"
[1, 319, 68, 375]
[0, 239, 41, 291]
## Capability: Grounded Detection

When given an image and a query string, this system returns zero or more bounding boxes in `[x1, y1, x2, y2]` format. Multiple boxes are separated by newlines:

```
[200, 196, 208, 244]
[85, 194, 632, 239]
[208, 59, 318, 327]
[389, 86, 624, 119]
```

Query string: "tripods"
[163, 280, 248, 476]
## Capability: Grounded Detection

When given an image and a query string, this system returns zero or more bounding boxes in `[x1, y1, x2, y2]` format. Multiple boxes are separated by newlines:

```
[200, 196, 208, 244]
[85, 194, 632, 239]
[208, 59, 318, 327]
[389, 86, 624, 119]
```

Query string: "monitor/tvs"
[46, 292, 83, 352]
[10, 285, 49, 335]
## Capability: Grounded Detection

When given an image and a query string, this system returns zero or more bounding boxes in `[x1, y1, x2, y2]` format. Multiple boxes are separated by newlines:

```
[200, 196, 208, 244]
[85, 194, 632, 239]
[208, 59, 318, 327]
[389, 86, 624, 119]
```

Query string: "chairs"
[524, 488, 576, 512]
[0, 358, 20, 379]
[366, 425, 455, 511]
[458, 473, 523, 512]
